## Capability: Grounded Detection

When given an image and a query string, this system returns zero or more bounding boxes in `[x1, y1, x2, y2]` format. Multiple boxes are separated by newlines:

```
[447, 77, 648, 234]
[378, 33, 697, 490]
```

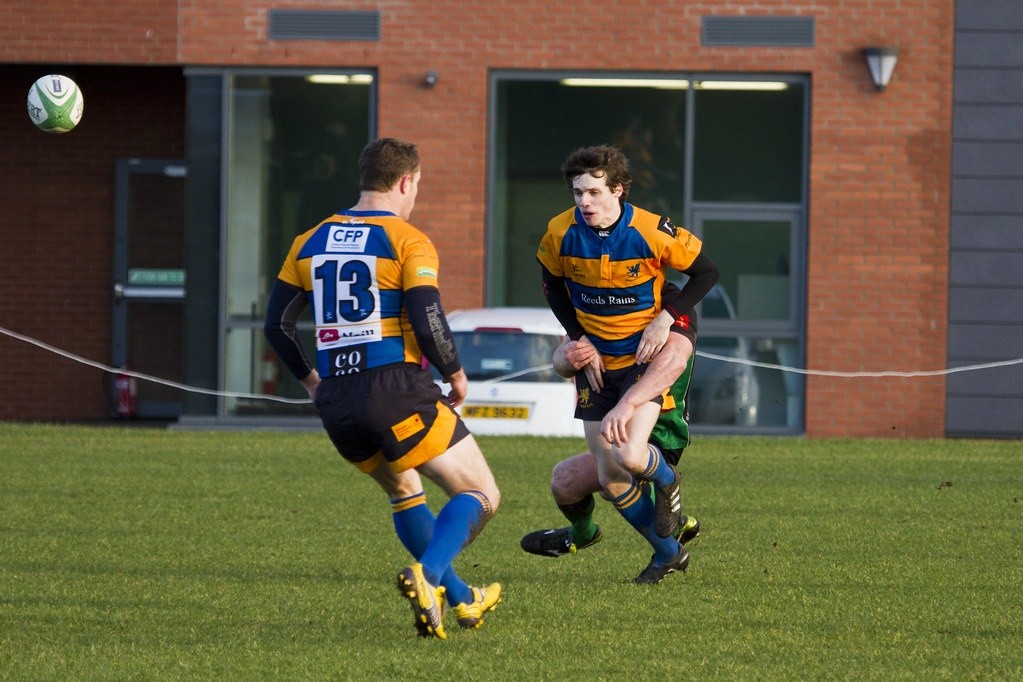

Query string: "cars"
[685, 284, 758, 426]
[433, 305, 583, 438]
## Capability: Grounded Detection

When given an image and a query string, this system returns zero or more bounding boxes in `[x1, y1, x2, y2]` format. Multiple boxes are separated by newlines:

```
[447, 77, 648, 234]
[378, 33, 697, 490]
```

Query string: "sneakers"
[396, 562, 446, 641]
[450, 582, 502, 630]
[653, 464, 681, 539]
[649, 514, 699, 562]
[519, 523, 604, 558]
[623, 542, 689, 585]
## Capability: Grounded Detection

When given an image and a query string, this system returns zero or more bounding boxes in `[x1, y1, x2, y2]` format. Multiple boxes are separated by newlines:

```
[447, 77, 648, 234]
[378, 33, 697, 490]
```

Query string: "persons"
[532, 145, 721, 585]
[519, 261, 699, 558]
[263, 137, 502, 638]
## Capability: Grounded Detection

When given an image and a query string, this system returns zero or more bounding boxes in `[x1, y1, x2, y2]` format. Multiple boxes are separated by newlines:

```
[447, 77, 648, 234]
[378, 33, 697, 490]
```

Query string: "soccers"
[25, 73, 84, 135]
